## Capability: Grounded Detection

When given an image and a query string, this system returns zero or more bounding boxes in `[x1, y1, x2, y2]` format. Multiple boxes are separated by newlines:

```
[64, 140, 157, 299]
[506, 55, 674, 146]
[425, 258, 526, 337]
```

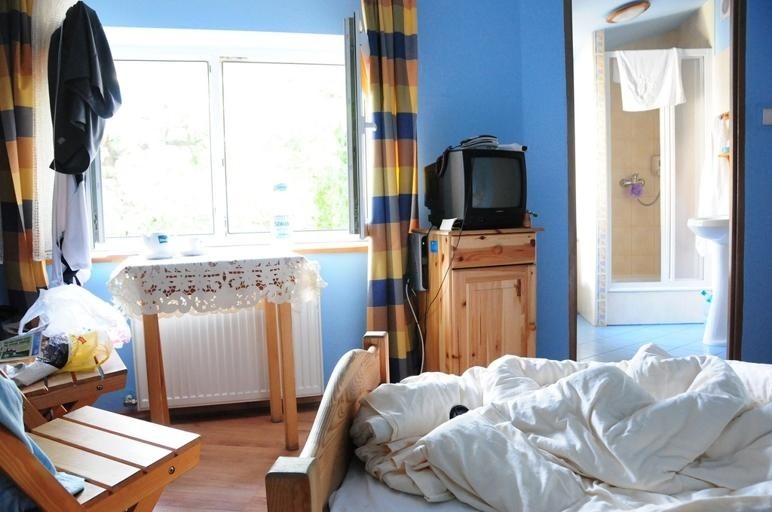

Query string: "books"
[0, 331, 42, 362]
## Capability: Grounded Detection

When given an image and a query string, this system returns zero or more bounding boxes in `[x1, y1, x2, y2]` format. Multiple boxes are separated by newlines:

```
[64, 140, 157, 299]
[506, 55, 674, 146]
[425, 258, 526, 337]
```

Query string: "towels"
[613, 46, 686, 112]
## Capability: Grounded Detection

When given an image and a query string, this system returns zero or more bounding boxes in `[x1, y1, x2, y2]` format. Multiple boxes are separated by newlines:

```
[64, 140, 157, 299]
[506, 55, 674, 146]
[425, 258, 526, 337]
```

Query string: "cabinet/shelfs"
[412, 227, 544, 377]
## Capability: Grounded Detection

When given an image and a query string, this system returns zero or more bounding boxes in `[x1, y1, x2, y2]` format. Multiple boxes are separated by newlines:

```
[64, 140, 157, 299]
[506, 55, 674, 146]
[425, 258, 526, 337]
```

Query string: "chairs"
[0, 339, 202, 512]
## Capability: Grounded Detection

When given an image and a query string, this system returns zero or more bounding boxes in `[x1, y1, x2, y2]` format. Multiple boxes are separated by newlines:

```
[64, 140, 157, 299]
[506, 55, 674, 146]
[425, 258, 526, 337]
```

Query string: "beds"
[265, 330, 772, 512]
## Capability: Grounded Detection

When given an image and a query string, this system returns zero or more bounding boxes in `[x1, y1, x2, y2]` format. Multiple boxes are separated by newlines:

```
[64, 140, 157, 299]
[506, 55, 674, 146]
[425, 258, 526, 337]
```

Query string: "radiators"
[124, 278, 325, 413]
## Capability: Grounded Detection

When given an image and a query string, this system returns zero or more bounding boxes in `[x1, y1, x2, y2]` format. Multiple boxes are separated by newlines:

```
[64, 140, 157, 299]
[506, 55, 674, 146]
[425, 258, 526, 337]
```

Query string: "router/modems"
[406, 233, 428, 292]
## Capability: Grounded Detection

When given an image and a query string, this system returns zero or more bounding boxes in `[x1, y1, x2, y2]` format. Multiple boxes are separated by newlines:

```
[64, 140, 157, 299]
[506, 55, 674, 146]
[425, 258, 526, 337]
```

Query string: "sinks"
[687, 218, 730, 238]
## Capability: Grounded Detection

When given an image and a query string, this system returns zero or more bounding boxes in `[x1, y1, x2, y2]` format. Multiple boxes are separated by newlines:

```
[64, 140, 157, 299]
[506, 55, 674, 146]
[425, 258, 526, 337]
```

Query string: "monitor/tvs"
[424, 149, 527, 231]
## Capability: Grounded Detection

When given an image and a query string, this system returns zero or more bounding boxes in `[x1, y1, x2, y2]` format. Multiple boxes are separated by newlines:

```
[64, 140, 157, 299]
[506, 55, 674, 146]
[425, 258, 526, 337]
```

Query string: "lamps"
[607, 0, 650, 23]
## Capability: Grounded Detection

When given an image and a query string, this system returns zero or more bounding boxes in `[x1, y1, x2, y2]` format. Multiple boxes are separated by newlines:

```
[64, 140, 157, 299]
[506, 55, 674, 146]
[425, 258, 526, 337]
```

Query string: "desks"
[105, 248, 328, 451]
